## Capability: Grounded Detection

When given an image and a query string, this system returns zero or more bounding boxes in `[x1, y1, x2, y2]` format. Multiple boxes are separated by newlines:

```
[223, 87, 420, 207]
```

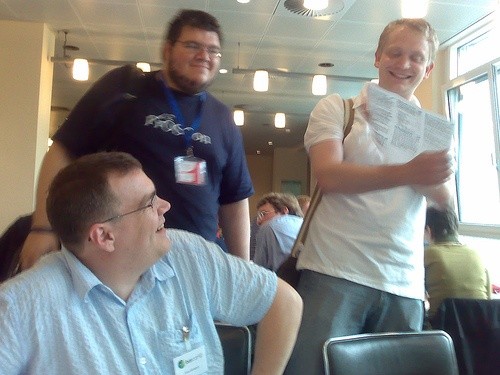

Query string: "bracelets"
[27, 226, 56, 236]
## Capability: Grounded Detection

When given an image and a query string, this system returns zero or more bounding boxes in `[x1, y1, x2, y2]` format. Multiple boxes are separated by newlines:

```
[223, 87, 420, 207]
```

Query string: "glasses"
[88, 193, 161, 242]
[175, 38, 223, 58]
[256, 208, 278, 226]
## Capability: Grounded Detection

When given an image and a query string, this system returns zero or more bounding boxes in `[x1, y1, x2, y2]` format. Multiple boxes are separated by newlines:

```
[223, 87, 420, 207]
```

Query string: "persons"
[424, 203, 490, 331]
[254, 192, 311, 271]
[285, 19, 454, 375]
[0, 213, 35, 284]
[19, 9, 256, 273]
[0, 152, 304, 375]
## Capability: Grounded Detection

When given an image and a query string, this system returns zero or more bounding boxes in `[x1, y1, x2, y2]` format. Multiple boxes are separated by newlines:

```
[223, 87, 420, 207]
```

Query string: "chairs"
[322, 330, 459, 375]
[435, 296, 500, 375]
[214, 322, 252, 375]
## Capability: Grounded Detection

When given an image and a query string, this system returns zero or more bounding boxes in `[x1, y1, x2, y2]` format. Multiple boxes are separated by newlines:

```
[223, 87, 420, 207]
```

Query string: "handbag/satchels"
[0, 214, 34, 284]
[276, 256, 301, 290]
